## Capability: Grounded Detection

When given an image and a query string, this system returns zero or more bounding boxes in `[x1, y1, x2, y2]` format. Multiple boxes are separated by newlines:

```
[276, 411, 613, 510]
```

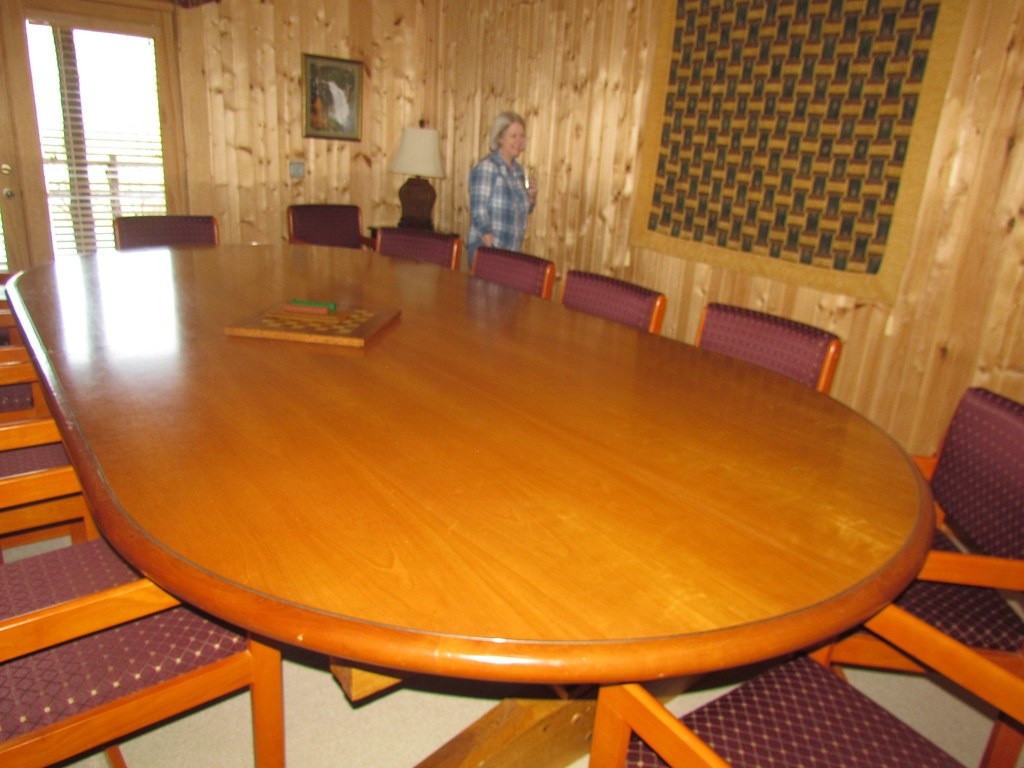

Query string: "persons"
[467, 111, 538, 267]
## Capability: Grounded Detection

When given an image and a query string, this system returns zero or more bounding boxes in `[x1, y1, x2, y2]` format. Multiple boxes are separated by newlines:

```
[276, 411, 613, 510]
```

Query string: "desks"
[0, 240, 936, 768]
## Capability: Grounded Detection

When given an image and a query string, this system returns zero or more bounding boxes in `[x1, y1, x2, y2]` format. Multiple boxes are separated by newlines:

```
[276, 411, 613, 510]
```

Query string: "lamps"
[388, 126, 447, 229]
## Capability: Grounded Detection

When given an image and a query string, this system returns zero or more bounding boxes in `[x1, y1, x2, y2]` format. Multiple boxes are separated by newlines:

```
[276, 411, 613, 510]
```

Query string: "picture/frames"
[302, 52, 363, 142]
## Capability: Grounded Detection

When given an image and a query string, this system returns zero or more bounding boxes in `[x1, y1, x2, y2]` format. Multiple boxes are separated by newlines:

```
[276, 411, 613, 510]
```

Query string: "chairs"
[0, 287, 287, 768]
[471, 246, 555, 299]
[376, 228, 461, 271]
[558, 270, 666, 333]
[592, 601, 1024, 768]
[694, 301, 842, 394]
[288, 205, 375, 252]
[113, 215, 220, 252]
[813, 385, 1024, 674]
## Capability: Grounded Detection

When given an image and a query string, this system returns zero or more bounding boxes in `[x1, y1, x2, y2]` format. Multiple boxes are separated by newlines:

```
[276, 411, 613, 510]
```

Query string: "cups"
[521, 167, 533, 189]
